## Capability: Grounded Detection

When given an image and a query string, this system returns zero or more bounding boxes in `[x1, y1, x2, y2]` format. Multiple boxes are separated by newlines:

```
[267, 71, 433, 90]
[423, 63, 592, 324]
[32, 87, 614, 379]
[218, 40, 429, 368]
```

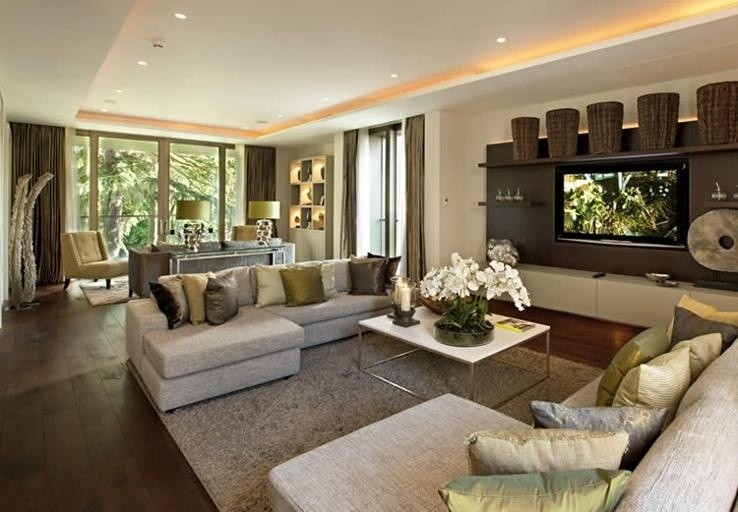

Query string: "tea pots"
[389, 277, 420, 327]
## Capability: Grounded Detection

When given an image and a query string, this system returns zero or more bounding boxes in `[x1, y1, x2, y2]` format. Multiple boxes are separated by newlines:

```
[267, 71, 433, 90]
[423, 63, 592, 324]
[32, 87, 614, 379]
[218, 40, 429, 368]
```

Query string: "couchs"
[265, 293, 736, 512]
[128, 238, 296, 299]
[59, 232, 129, 290]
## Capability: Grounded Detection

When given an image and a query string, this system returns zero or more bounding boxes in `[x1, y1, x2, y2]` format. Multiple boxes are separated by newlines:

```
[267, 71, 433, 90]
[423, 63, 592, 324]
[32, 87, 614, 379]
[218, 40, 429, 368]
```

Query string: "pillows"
[671, 331, 724, 382]
[439, 470, 632, 512]
[469, 429, 628, 474]
[530, 397, 667, 465]
[613, 347, 690, 427]
[594, 327, 671, 407]
[669, 293, 737, 355]
[149, 254, 403, 329]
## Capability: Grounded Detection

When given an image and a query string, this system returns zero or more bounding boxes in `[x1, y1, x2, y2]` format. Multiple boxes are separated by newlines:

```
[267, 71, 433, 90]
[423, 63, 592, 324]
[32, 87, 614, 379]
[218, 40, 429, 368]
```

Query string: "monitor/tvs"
[555, 158, 691, 251]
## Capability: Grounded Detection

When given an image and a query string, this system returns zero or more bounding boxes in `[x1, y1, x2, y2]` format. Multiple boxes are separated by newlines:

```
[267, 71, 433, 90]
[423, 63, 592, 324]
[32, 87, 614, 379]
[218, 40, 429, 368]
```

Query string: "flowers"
[421, 251, 531, 331]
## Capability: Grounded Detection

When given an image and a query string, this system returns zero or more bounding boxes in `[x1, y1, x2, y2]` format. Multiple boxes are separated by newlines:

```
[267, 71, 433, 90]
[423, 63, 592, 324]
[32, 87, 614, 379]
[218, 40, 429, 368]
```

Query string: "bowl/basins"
[646, 272, 669, 281]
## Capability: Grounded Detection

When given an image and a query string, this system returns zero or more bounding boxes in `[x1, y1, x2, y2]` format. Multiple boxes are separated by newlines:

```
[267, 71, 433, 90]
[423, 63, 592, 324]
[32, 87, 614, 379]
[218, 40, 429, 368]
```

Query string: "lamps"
[176, 200, 210, 254]
[248, 200, 280, 248]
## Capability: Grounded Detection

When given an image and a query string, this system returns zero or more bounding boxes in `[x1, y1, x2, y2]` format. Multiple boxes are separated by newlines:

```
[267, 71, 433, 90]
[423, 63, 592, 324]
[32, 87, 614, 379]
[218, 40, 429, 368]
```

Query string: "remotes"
[592, 271, 607, 278]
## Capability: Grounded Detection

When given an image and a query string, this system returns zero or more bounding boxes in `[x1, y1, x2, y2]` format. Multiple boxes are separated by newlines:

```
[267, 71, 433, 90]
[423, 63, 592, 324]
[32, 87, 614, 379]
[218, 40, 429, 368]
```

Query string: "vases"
[435, 319, 493, 346]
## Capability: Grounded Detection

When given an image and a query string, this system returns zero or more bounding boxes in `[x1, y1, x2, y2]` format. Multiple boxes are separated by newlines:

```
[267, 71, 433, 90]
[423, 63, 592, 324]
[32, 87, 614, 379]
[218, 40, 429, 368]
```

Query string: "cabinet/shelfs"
[289, 156, 332, 260]
[476, 140, 737, 205]
[475, 260, 737, 329]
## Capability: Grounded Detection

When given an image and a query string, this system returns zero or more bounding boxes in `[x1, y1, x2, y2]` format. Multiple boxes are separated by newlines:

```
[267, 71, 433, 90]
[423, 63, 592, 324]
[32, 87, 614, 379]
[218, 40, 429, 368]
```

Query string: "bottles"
[709, 180, 737, 200]
[494, 187, 523, 201]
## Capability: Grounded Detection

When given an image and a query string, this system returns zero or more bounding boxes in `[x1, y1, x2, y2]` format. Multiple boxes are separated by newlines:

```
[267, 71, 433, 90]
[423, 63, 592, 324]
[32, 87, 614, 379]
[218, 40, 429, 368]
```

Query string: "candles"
[393, 279, 419, 311]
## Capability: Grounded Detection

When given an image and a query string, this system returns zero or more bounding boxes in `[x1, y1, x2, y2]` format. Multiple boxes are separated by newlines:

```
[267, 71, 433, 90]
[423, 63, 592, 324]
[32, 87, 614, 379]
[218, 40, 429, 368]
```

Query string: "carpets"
[79, 273, 137, 307]
[125, 332, 606, 512]
